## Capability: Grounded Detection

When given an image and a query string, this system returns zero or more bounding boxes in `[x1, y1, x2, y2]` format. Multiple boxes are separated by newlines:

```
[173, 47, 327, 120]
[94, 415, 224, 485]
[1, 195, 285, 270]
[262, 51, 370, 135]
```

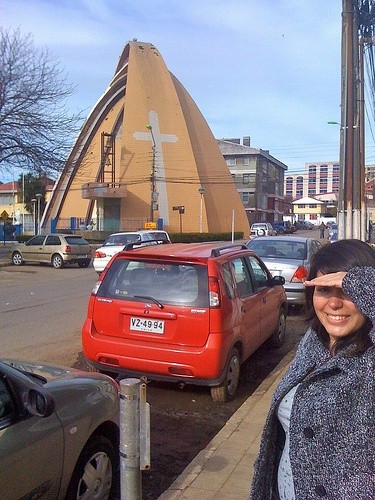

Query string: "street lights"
[31, 198, 36, 235]
[199, 186, 205, 233]
[36, 194, 42, 235]
[145, 125, 156, 222]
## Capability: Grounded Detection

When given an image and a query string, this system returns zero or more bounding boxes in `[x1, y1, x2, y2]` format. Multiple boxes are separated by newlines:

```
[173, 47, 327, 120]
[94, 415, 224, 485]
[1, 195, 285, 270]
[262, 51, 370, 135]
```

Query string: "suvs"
[93, 229, 172, 276]
[250, 222, 278, 237]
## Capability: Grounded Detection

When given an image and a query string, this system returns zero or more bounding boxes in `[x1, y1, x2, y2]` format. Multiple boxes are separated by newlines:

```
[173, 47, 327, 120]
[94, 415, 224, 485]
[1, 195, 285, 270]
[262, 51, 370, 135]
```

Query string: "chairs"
[264, 246, 276, 255]
[130, 268, 154, 287]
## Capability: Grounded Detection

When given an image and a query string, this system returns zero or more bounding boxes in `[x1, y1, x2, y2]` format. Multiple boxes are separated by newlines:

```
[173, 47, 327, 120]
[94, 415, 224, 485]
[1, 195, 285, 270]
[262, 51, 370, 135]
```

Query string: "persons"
[319, 222, 325, 239]
[249, 239, 375, 500]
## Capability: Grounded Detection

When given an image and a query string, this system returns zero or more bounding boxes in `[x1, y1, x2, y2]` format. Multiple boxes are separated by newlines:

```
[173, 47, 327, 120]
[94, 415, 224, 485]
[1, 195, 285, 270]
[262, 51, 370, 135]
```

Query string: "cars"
[325, 220, 338, 244]
[6, 233, 93, 269]
[0, 358, 120, 500]
[232, 236, 324, 316]
[81, 238, 289, 404]
[269, 220, 315, 235]
